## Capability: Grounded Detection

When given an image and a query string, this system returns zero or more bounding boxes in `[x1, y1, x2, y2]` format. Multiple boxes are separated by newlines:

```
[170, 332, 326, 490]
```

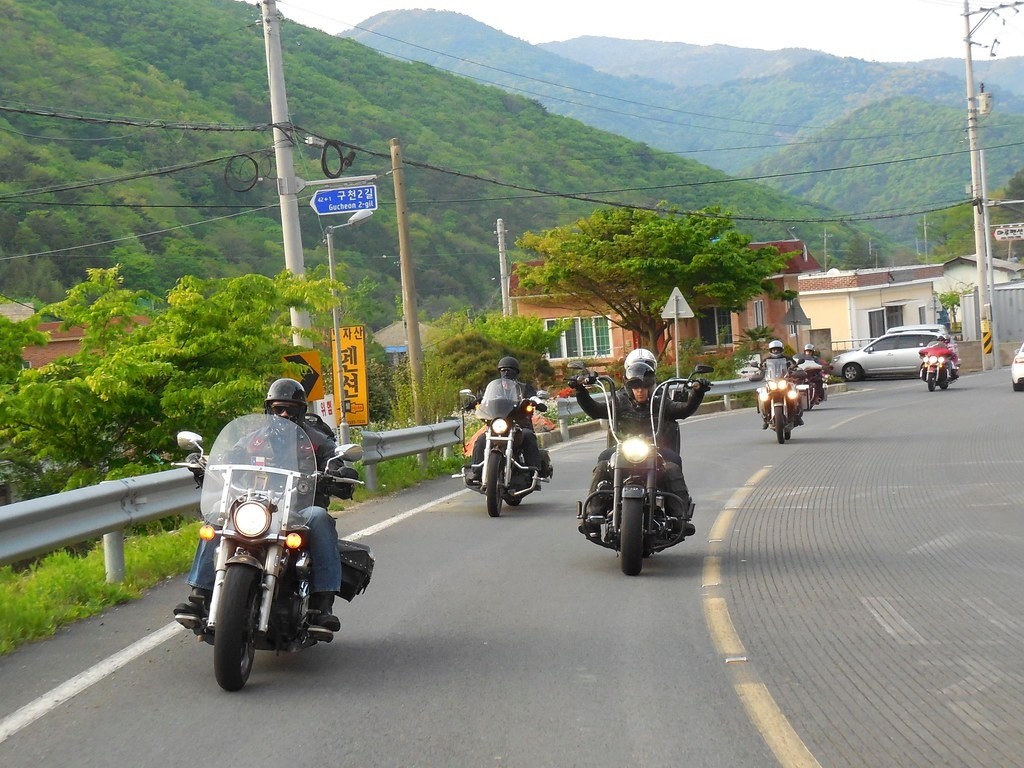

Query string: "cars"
[1012, 342, 1024, 392]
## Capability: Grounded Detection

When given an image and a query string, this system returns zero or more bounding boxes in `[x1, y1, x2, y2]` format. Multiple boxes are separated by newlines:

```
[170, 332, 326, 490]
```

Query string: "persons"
[754, 340, 804, 430]
[174, 378, 358, 633]
[798, 343, 834, 402]
[462, 357, 547, 482]
[568, 348, 710, 536]
[920, 334, 959, 380]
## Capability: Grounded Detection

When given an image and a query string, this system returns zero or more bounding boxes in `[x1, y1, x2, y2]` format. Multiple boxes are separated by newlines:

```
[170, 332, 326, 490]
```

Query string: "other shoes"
[665, 516, 696, 537]
[578, 517, 605, 534]
[797, 418, 804, 426]
[762, 423, 769, 430]
[466, 476, 482, 485]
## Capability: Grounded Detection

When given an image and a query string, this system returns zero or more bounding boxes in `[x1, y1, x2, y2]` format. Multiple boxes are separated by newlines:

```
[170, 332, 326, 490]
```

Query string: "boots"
[951, 369, 959, 378]
[173, 587, 212, 618]
[311, 594, 341, 632]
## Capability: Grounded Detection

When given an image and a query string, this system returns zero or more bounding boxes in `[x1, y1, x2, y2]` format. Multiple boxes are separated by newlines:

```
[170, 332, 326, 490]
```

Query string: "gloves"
[754, 370, 762, 375]
[326, 466, 359, 488]
[536, 403, 547, 413]
[185, 453, 206, 472]
[794, 367, 803, 371]
[567, 374, 585, 391]
[696, 376, 712, 393]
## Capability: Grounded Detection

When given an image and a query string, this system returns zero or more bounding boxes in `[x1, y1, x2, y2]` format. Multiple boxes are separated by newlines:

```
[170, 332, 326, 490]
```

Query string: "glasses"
[938, 340, 944, 341]
[269, 405, 302, 417]
[500, 368, 516, 372]
[628, 381, 651, 389]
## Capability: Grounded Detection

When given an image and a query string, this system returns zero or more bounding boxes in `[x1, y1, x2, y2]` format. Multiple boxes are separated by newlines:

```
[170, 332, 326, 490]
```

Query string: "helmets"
[769, 340, 784, 358]
[497, 357, 521, 375]
[624, 349, 658, 385]
[804, 344, 815, 351]
[265, 378, 310, 406]
[937, 334, 952, 342]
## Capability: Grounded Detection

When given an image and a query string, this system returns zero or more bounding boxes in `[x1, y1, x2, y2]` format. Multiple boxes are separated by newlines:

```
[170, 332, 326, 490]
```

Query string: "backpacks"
[298, 412, 336, 444]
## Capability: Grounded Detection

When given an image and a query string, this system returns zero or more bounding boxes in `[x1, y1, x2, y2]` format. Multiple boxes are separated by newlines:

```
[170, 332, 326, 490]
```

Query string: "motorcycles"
[170, 413, 365, 691]
[452, 378, 550, 518]
[920, 340, 952, 392]
[563, 360, 714, 577]
[750, 353, 827, 444]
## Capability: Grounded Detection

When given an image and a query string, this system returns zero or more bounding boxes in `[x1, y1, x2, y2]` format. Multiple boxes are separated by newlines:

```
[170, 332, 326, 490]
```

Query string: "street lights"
[325, 210, 373, 466]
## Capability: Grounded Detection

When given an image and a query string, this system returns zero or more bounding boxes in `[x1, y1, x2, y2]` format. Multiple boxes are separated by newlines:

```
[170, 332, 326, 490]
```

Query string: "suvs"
[828, 324, 962, 382]
